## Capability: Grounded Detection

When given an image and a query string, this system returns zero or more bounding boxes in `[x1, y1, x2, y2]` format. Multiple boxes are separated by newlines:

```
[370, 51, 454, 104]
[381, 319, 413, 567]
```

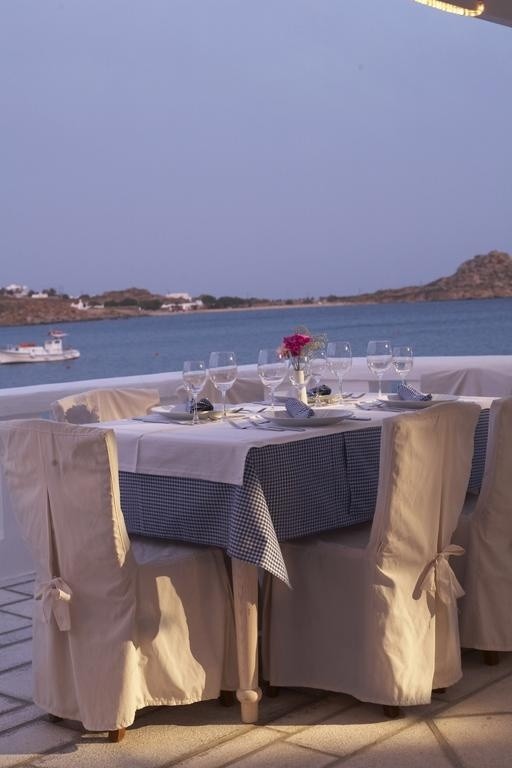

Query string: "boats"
[0, 329, 80, 362]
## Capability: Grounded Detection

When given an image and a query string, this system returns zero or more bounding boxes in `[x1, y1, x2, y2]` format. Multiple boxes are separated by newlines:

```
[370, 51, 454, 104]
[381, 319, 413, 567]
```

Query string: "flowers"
[277, 325, 327, 372]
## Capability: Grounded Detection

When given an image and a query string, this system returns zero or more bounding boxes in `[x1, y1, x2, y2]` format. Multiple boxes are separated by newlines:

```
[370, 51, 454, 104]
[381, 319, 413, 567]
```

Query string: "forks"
[225, 416, 305, 432]
[240, 407, 267, 413]
[341, 391, 367, 399]
[225, 406, 243, 414]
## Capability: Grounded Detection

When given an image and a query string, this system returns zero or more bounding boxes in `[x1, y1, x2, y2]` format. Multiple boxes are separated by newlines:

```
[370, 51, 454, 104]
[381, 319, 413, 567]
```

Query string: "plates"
[376, 393, 459, 408]
[259, 408, 353, 426]
[150, 403, 243, 421]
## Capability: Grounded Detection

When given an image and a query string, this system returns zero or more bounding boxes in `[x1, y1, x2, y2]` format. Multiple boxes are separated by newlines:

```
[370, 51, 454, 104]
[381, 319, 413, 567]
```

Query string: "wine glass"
[365, 339, 391, 403]
[391, 345, 412, 387]
[257, 347, 286, 420]
[307, 346, 327, 406]
[207, 349, 242, 424]
[287, 354, 313, 409]
[326, 339, 354, 408]
[182, 358, 208, 426]
